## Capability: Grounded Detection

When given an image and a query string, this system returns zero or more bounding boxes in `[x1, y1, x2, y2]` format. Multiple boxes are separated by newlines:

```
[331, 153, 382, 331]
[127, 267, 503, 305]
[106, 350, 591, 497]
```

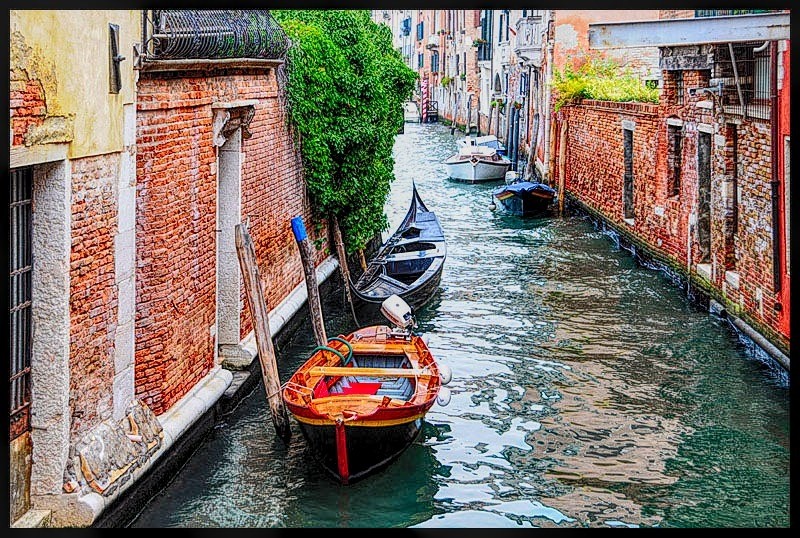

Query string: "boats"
[401, 102, 421, 123]
[346, 177, 448, 317]
[441, 134, 512, 183]
[282, 294, 452, 486]
[493, 181, 556, 217]
